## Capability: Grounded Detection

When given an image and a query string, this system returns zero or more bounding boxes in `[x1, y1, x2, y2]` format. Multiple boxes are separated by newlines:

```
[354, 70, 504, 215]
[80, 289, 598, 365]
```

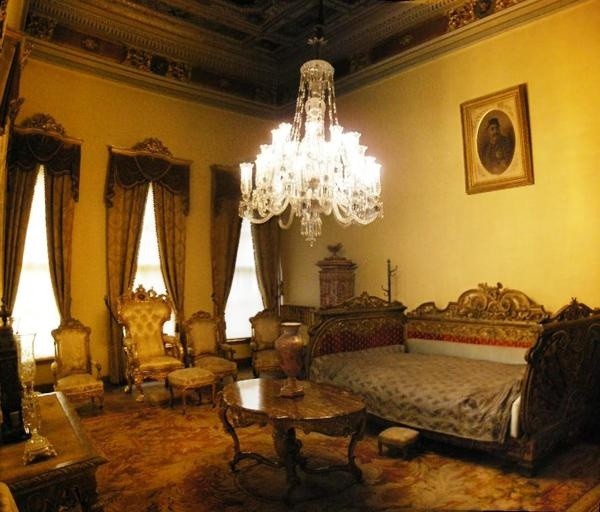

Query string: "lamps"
[238, 2, 385, 248]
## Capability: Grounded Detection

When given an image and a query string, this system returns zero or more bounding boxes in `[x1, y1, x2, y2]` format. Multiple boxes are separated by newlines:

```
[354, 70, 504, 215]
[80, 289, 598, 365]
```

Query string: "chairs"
[249, 309, 283, 378]
[115, 284, 189, 403]
[50, 318, 105, 409]
[185, 310, 239, 399]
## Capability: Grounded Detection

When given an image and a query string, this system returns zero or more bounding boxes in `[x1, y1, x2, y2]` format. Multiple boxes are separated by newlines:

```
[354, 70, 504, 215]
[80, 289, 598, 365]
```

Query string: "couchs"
[305, 283, 600, 476]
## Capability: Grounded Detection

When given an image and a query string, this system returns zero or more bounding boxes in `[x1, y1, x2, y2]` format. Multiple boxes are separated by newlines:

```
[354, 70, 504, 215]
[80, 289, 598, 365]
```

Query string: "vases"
[275, 321, 308, 399]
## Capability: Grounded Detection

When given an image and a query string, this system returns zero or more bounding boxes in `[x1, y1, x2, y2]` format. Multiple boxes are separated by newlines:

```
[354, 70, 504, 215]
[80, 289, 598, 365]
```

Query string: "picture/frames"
[458, 83, 535, 195]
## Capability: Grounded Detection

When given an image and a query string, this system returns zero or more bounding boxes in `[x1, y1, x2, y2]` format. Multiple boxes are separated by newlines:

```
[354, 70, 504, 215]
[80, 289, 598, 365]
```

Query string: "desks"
[0, 392, 111, 510]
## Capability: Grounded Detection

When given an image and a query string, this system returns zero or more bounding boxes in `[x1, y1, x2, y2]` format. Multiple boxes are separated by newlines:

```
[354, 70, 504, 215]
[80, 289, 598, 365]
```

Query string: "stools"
[165, 368, 218, 414]
[377, 426, 421, 462]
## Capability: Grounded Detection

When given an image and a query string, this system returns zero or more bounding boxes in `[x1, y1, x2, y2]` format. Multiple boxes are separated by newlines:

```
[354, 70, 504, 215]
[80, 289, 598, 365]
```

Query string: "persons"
[482, 117, 513, 173]
